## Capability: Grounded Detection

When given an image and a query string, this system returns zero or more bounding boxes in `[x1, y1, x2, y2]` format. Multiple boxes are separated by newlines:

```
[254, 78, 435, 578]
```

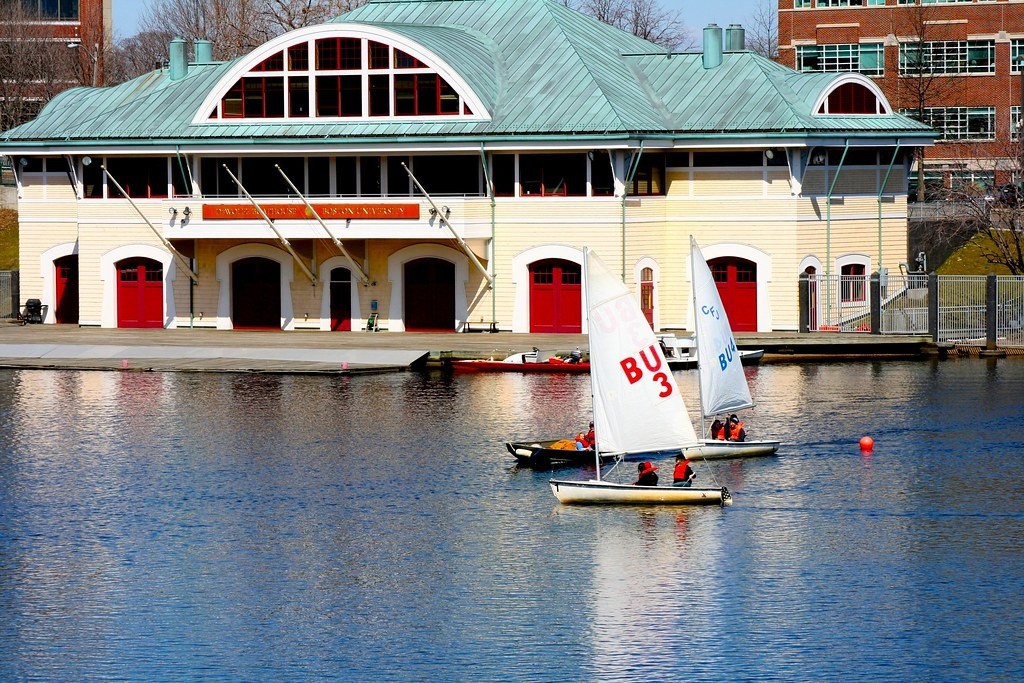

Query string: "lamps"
[347, 218, 351, 223]
[169, 206, 193, 224]
[270, 218, 275, 223]
[429, 206, 451, 223]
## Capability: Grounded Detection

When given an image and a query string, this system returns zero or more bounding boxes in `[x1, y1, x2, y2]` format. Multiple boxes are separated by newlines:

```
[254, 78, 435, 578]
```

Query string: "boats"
[450, 333, 764, 372]
[507, 441, 600, 471]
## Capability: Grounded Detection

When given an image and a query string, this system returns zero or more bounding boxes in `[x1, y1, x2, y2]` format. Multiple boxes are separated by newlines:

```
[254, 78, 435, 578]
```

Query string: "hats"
[730, 417, 739, 424]
[588, 421, 594, 426]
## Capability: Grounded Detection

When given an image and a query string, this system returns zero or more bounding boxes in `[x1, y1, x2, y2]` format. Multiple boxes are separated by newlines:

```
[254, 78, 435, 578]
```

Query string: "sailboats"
[549, 245, 727, 507]
[677, 234, 781, 460]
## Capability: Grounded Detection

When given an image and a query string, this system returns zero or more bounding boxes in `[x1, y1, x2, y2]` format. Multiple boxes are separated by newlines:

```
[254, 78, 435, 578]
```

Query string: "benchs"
[459, 322, 499, 333]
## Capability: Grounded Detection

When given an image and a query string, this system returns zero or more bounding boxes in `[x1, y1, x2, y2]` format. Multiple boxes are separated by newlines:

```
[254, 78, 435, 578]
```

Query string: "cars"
[984, 184, 1022, 206]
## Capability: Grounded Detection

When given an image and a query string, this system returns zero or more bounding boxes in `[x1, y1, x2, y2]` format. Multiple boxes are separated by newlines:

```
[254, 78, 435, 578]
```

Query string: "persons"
[574, 421, 595, 451]
[711, 414, 746, 442]
[672, 452, 696, 488]
[633, 463, 660, 486]
